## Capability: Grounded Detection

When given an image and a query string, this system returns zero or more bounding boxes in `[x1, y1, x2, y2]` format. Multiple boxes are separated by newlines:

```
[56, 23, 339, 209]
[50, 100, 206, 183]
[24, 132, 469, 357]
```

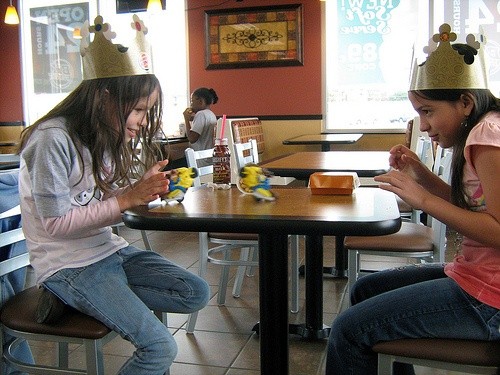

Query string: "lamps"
[4, 0, 19, 25]
[73, 28, 82, 40]
[147, 0, 162, 12]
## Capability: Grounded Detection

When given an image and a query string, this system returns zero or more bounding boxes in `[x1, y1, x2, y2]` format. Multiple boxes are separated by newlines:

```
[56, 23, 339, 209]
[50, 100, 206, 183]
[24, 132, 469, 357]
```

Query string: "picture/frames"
[203, 3, 304, 70]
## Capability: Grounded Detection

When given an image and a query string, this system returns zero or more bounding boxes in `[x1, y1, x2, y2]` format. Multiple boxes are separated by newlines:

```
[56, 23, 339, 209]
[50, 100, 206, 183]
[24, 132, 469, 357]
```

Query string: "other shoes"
[35, 287, 65, 323]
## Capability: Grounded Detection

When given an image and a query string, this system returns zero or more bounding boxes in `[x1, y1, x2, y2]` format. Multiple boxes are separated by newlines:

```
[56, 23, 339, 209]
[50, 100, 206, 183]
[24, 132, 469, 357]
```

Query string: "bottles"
[213, 138, 231, 185]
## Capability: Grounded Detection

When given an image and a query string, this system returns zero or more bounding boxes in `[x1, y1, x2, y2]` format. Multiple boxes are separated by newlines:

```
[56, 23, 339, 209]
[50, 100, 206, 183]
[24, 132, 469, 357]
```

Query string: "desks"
[122, 185, 402, 375]
[260, 151, 392, 280]
[282, 133, 363, 152]
[160, 137, 189, 171]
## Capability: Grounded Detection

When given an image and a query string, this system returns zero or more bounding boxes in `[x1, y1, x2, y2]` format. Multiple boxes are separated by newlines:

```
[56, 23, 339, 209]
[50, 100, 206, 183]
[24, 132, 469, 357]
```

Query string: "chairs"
[0, 153, 170, 375]
[344, 137, 500, 375]
[184, 148, 298, 334]
[232, 139, 258, 298]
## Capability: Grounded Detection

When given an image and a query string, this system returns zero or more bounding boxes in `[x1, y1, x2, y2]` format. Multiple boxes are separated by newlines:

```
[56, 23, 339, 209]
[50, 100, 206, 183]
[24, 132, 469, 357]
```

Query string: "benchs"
[405, 117, 432, 153]
[212, 117, 265, 157]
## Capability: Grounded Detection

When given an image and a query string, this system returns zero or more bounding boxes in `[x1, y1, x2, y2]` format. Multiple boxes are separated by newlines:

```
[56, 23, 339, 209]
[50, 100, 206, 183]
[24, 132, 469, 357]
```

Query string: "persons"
[161, 87, 219, 172]
[325, 44, 500, 375]
[14, 46, 210, 375]
